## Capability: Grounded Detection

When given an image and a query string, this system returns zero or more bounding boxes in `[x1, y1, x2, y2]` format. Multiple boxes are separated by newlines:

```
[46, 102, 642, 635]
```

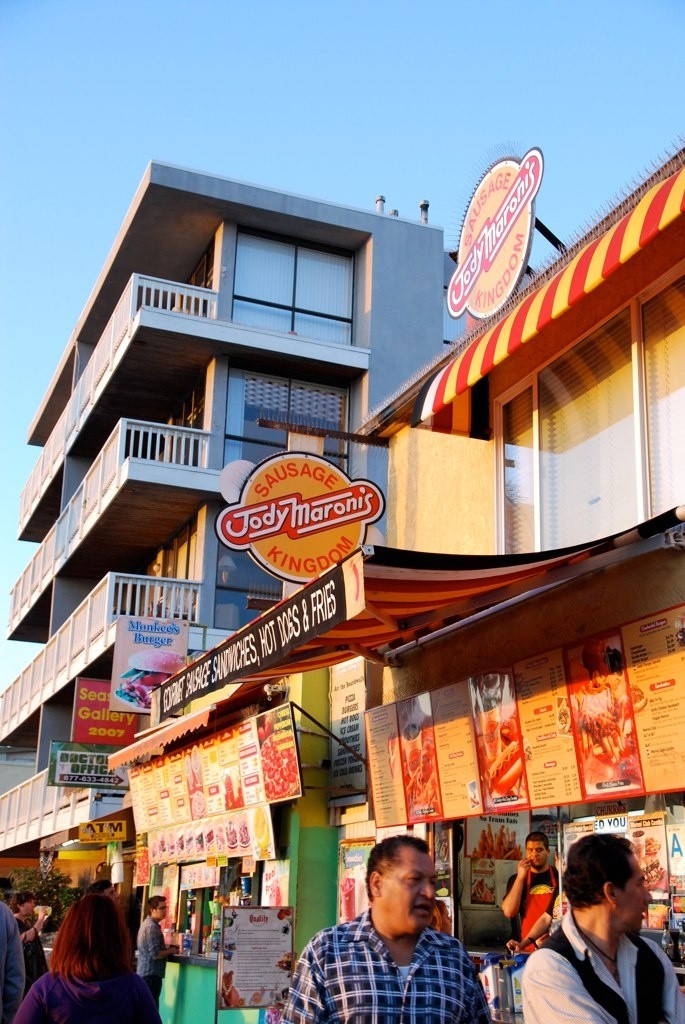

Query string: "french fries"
[574, 684, 628, 763]
[472, 823, 522, 861]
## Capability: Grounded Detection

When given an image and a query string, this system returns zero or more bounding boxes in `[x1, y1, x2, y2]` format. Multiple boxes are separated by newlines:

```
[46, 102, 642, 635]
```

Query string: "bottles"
[661, 920, 674, 962]
[202, 931, 208, 954]
[182, 929, 192, 956]
[677, 921, 685, 963]
[33, 905, 52, 915]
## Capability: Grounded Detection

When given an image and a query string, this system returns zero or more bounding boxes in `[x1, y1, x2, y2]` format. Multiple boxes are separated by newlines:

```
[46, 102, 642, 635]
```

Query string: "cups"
[240, 876, 251, 897]
[185, 744, 201, 787]
[479, 706, 501, 759]
[402, 724, 423, 778]
[341, 878, 355, 921]
[632, 830, 645, 858]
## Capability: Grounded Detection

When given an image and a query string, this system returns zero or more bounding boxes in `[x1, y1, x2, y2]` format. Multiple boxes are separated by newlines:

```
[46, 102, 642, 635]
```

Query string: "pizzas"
[260, 730, 300, 801]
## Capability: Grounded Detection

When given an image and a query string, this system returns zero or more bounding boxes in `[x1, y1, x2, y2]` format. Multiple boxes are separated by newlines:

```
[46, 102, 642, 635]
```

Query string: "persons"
[0, 901, 26, 1024]
[277, 835, 494, 1024]
[11, 890, 49, 1003]
[13, 893, 162, 1024]
[500, 830, 685, 1024]
[136, 896, 180, 1013]
[86, 879, 122, 904]
[436, 899, 452, 936]
[429, 904, 442, 931]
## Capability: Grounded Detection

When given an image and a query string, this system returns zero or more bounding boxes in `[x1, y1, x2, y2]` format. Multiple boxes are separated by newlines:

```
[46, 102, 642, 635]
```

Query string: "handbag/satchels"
[23, 929, 40, 958]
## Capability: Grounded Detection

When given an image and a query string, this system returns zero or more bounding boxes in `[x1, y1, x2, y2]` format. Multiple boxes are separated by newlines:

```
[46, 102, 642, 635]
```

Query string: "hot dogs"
[488, 741, 522, 796]
[406, 755, 436, 811]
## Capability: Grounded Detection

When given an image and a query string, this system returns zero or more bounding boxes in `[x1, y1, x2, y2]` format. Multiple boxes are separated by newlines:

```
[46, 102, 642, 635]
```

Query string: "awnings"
[39, 806, 135, 851]
[107, 675, 285, 774]
[408, 165, 685, 426]
[145, 503, 685, 727]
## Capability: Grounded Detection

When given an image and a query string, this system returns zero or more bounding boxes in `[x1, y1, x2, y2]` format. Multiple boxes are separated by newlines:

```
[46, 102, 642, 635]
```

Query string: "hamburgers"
[115, 649, 187, 710]
[644, 856, 664, 889]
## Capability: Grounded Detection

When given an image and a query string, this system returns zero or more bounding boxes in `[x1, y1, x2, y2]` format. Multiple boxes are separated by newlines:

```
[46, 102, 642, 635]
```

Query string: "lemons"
[252, 807, 269, 851]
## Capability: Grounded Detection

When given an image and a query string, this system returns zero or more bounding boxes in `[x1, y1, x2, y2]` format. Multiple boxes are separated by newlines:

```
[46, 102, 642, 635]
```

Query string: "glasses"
[154, 906, 167, 909]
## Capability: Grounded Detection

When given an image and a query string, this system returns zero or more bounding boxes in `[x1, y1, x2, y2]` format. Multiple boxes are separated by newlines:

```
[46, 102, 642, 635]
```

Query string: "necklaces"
[577, 928, 620, 984]
